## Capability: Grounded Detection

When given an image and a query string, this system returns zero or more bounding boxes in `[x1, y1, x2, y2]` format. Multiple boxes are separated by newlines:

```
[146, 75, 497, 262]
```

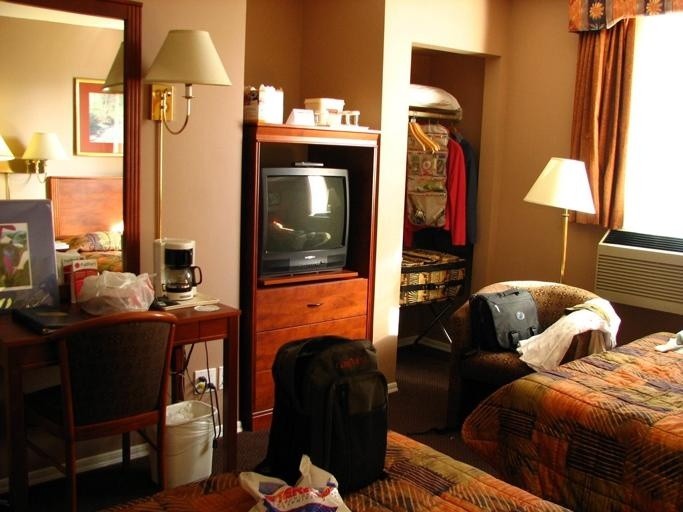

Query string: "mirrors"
[0, 0, 140, 304]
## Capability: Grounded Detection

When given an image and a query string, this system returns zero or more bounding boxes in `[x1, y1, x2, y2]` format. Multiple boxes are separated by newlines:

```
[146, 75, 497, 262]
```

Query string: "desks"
[0, 302, 239, 512]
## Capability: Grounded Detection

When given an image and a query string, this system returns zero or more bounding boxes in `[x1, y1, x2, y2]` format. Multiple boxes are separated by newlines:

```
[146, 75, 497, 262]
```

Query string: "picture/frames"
[72, 77, 122, 157]
[0, 199, 61, 311]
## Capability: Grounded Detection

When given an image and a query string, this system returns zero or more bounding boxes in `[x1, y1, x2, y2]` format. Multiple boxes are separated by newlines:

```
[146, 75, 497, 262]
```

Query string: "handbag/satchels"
[237, 453, 353, 512]
[465, 287, 542, 354]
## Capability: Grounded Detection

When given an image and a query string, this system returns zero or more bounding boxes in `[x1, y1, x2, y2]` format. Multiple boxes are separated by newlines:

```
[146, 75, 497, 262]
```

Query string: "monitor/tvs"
[259, 167, 350, 278]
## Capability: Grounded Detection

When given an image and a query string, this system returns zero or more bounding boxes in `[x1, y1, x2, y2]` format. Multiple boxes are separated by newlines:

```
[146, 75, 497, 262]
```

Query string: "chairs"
[445, 276, 614, 431]
[51, 309, 177, 512]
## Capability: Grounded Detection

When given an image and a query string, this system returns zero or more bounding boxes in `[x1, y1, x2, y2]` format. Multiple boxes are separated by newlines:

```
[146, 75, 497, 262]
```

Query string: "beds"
[44, 174, 126, 282]
[104, 421, 571, 511]
[456, 329, 682, 512]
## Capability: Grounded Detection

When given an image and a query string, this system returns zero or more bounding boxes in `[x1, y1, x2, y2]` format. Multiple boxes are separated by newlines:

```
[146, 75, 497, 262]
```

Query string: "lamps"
[102, 40, 124, 94]
[148, 28, 232, 136]
[0, 123, 53, 184]
[521, 153, 598, 280]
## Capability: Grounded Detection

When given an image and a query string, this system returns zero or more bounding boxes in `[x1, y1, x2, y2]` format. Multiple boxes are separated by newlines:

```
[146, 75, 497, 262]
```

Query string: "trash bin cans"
[151, 400, 218, 491]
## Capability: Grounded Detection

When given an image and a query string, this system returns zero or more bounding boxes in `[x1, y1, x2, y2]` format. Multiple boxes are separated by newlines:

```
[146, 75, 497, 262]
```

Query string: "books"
[11, 305, 87, 335]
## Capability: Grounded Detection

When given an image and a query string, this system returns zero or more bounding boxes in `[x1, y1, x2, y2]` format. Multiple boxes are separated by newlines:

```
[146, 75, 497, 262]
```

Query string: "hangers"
[407, 108, 456, 155]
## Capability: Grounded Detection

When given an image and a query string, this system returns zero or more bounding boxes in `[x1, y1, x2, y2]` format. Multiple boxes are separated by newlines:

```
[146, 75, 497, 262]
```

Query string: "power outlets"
[193, 367, 216, 394]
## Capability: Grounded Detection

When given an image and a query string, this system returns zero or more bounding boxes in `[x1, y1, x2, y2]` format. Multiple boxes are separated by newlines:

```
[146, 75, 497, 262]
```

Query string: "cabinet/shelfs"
[398, 245, 466, 353]
[242, 121, 380, 436]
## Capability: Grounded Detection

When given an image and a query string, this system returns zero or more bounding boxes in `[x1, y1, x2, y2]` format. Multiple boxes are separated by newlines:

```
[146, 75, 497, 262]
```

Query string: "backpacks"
[254, 335, 391, 493]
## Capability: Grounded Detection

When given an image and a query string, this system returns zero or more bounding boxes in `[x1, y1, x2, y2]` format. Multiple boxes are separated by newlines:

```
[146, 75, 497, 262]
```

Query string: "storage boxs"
[303, 97, 344, 125]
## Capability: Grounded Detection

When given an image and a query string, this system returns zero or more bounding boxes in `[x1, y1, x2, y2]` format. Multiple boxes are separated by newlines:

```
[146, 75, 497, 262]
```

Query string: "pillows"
[64, 226, 122, 255]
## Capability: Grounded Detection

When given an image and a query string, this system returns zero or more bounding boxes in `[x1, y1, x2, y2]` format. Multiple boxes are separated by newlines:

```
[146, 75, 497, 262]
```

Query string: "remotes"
[292, 161, 324, 167]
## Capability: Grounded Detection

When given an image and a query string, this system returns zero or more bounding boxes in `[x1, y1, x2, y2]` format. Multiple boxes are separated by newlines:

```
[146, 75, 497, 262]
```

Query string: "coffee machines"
[152, 237, 203, 302]
